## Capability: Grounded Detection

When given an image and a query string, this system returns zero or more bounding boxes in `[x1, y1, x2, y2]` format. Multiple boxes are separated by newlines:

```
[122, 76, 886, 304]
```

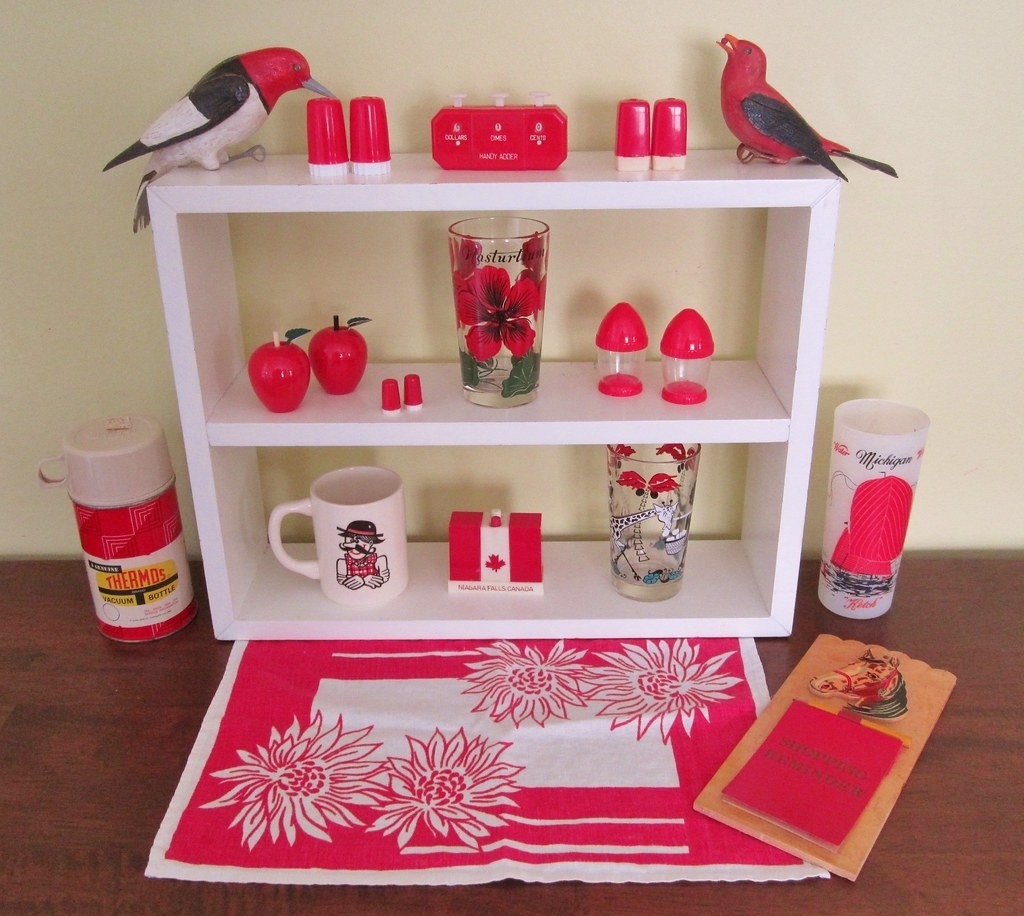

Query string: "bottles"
[659, 307, 715, 407]
[36, 412, 198, 645]
[595, 300, 649, 398]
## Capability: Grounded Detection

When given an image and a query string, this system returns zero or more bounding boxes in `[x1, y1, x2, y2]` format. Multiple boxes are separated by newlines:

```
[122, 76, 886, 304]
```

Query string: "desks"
[0, 552, 1024, 916]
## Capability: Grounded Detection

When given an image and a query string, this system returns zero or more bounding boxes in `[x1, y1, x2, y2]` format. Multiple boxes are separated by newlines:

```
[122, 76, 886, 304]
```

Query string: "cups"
[447, 216, 553, 410]
[604, 443, 702, 603]
[268, 465, 409, 612]
[819, 397, 931, 622]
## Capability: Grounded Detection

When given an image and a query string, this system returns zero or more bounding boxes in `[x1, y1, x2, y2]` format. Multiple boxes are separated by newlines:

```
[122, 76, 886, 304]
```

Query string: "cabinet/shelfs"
[145, 150, 839, 642]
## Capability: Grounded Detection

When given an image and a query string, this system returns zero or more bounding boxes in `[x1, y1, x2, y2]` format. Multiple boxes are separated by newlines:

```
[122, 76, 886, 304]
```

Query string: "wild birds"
[100, 48, 337, 233]
[716, 33, 899, 183]
